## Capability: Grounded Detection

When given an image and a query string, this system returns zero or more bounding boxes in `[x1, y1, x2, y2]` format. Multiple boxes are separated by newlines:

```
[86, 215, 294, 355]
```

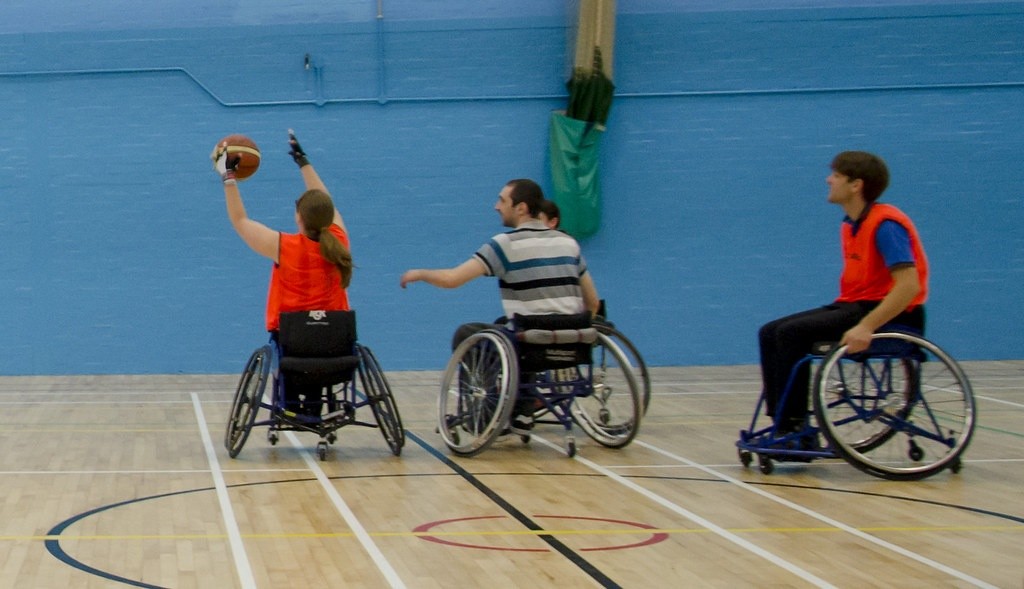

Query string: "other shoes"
[484, 398, 534, 435]
[749, 427, 819, 450]
[285, 399, 302, 414]
[302, 399, 323, 419]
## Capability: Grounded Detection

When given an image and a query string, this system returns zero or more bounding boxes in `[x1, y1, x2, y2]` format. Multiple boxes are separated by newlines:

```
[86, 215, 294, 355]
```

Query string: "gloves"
[211, 142, 242, 185]
[287, 134, 310, 169]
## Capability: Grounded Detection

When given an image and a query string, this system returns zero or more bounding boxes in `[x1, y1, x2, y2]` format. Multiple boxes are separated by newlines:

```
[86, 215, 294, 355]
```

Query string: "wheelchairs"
[733, 329, 978, 480]
[224, 308, 405, 458]
[436, 296, 653, 461]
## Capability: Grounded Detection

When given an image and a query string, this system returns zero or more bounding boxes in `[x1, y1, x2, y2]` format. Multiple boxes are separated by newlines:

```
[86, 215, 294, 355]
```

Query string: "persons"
[400, 178, 601, 428]
[211, 128, 352, 415]
[758, 150, 928, 457]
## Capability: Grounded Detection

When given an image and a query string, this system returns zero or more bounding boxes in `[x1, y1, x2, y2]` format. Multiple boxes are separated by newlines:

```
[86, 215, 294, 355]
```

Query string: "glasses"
[293, 196, 302, 211]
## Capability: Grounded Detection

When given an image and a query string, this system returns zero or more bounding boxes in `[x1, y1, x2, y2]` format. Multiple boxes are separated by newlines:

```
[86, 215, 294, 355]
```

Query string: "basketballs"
[216, 135, 260, 181]
